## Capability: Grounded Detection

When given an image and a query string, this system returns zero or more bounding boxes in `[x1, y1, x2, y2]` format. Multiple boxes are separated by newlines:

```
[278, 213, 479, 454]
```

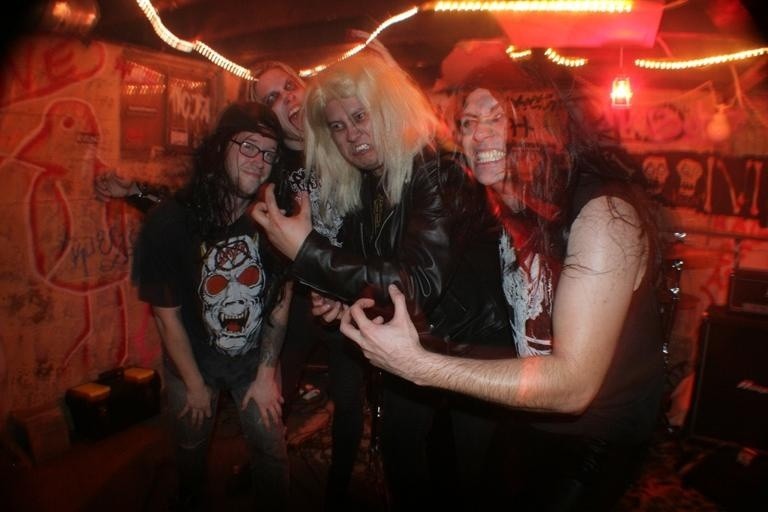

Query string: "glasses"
[229, 137, 280, 166]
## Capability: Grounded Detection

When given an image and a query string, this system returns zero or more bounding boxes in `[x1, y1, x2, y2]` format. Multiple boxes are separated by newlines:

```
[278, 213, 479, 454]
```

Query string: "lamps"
[609, 44, 633, 109]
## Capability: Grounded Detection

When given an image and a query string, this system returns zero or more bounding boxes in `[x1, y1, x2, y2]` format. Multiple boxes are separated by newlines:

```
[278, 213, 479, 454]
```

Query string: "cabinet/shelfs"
[687, 301, 767, 454]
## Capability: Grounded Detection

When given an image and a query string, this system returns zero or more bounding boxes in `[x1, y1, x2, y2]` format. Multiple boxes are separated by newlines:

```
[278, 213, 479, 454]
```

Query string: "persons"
[92, 59, 367, 511]
[339, 54, 671, 509]
[245, 49, 515, 511]
[138, 102, 298, 512]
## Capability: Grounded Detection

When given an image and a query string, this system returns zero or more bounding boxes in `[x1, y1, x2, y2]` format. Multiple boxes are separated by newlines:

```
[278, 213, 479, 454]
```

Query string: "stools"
[121, 366, 160, 419]
[9, 405, 71, 465]
[66, 382, 112, 436]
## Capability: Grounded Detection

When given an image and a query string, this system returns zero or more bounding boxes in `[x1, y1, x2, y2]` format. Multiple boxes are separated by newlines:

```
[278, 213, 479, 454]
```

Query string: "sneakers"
[222, 461, 254, 498]
